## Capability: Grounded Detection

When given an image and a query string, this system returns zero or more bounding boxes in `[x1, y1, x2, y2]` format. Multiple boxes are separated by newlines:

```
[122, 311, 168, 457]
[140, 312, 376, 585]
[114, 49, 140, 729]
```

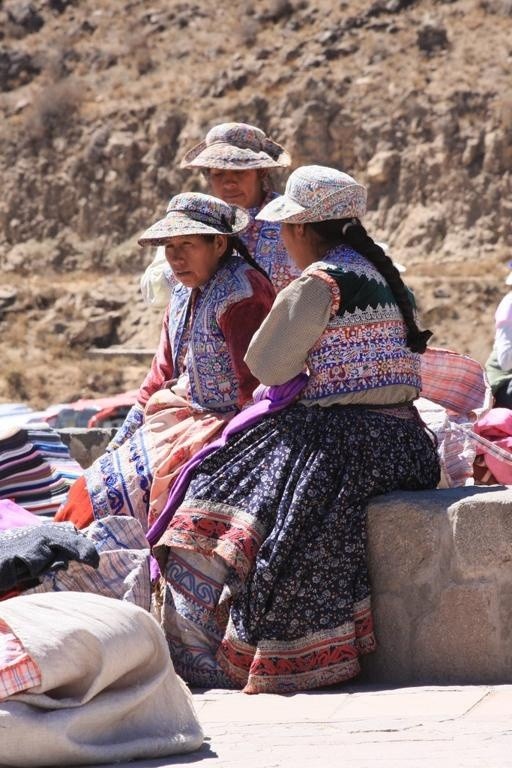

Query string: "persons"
[179, 122, 304, 295]
[53, 190, 278, 535]
[483, 261, 512, 411]
[145, 162, 440, 694]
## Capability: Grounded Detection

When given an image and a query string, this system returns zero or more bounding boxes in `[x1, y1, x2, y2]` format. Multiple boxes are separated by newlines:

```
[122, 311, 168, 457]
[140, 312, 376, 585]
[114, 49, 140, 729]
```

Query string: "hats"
[253, 165, 367, 225]
[179, 122, 292, 172]
[138, 192, 251, 249]
